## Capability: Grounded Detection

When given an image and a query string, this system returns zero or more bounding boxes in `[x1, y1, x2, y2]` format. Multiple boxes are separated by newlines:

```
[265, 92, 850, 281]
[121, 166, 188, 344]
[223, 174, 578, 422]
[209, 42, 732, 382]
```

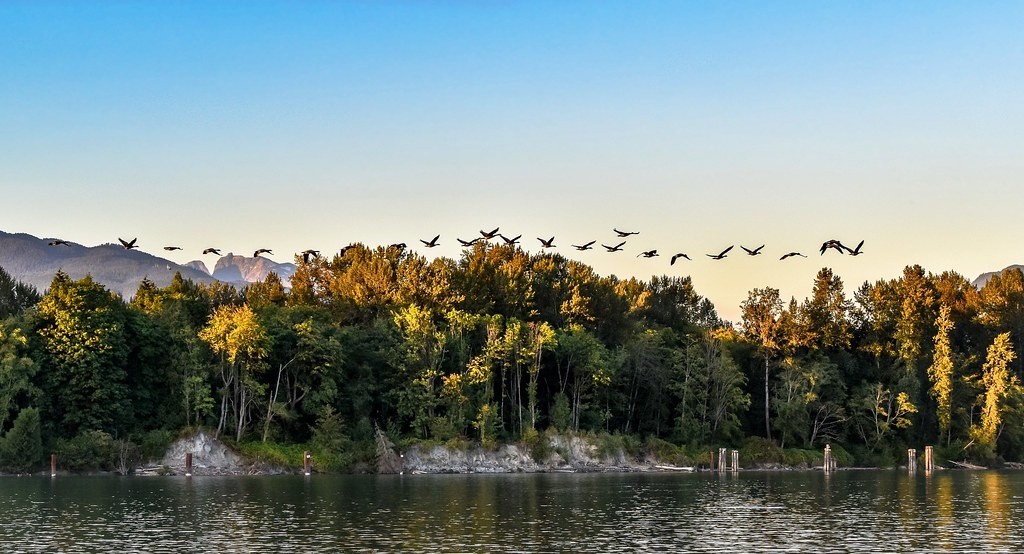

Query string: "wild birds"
[48, 229, 865, 267]
